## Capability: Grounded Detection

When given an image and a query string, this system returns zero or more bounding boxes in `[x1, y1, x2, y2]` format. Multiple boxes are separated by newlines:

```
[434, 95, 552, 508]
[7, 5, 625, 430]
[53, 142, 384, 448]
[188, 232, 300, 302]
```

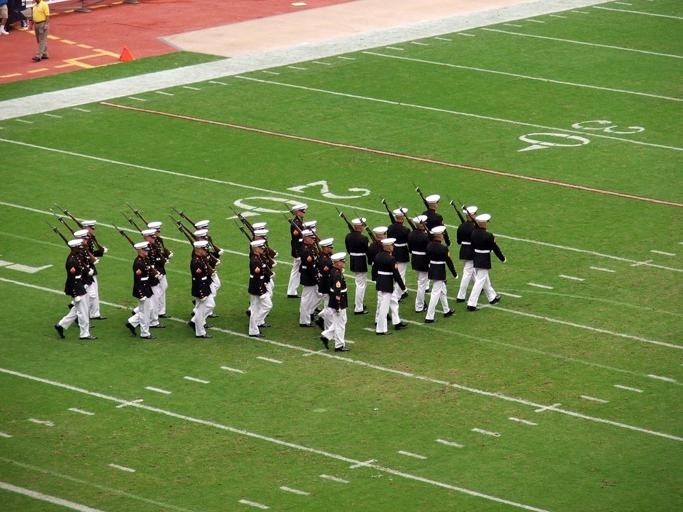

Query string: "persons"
[126, 222, 174, 339]
[0, 0, 34, 35]
[246, 222, 277, 338]
[345, 194, 507, 334]
[188, 220, 225, 339]
[55, 220, 107, 340]
[32, 0, 50, 62]
[288, 203, 351, 351]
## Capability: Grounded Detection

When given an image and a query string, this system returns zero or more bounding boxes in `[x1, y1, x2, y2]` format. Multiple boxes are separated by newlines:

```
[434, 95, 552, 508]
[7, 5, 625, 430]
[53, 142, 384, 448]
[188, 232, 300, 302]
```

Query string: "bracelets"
[44, 24, 48, 30]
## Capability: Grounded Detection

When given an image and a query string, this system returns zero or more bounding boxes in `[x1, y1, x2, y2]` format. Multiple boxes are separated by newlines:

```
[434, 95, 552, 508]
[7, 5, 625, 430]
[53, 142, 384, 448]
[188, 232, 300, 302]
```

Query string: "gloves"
[74, 250, 278, 302]
[456, 276, 458, 279]
[504, 256, 506, 263]
[341, 268, 345, 273]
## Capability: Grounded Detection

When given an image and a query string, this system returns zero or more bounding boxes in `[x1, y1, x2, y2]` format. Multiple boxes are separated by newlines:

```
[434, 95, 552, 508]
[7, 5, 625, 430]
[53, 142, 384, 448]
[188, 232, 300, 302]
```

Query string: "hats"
[292, 194, 491, 262]
[67, 219, 269, 250]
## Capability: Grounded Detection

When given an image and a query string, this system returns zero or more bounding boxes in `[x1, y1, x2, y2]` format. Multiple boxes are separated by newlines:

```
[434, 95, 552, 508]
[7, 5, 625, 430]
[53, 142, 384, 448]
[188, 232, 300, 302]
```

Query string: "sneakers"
[42, 56, 48, 59]
[32, 57, 40, 61]
[0, 30, 10, 35]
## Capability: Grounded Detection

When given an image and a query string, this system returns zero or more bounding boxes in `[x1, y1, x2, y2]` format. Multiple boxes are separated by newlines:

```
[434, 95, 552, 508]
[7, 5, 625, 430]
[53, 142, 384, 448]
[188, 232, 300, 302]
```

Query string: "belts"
[35, 21, 46, 23]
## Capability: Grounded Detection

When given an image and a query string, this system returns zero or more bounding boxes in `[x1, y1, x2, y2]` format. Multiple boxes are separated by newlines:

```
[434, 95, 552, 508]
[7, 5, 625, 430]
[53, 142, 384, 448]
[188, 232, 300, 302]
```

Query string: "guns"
[44, 203, 103, 285]
[414, 183, 481, 233]
[113, 201, 170, 277]
[333, 206, 383, 250]
[169, 206, 220, 276]
[380, 201, 432, 243]
[228, 206, 276, 277]
[283, 203, 321, 257]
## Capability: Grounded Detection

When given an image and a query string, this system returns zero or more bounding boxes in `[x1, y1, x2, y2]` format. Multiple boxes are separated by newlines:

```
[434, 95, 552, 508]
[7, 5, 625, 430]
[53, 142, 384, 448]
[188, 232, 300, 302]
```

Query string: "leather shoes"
[54, 299, 271, 340]
[287, 280, 501, 352]
